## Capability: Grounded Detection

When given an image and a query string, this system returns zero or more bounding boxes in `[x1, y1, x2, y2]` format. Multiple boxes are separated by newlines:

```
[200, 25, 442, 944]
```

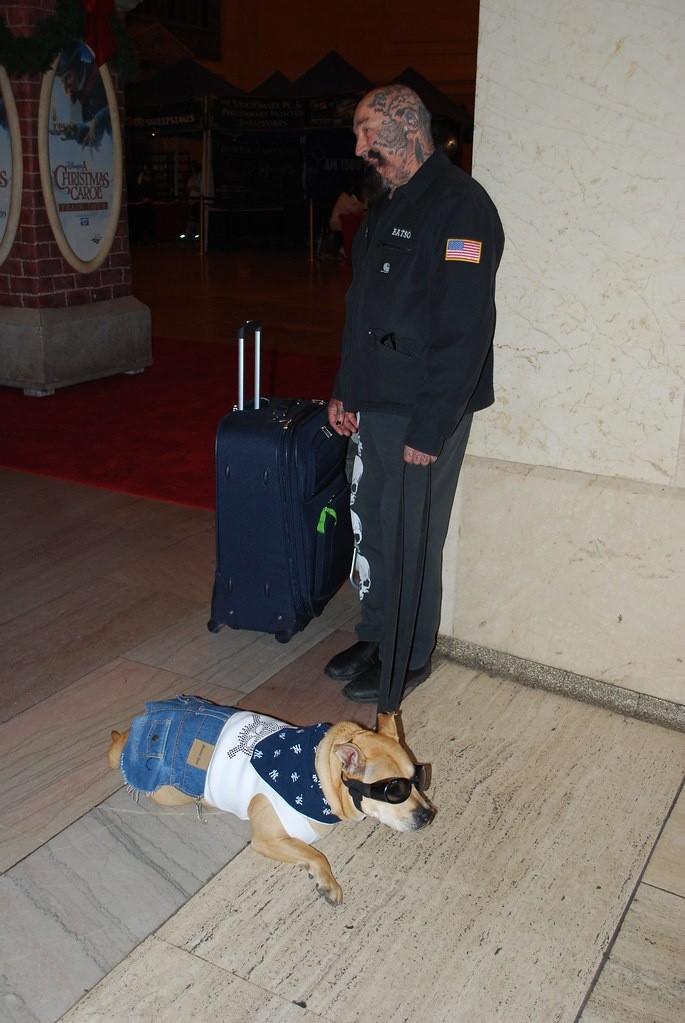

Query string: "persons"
[184, 160, 202, 234]
[324, 83, 505, 703]
[330, 180, 364, 257]
[127, 168, 157, 247]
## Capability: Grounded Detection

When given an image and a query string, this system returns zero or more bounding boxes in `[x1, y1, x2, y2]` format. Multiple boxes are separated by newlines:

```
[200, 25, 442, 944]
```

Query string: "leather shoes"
[341, 655, 431, 704]
[324, 641, 379, 681]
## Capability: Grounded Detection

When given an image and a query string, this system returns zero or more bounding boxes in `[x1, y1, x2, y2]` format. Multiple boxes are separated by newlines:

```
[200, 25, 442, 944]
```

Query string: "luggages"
[206, 320, 355, 645]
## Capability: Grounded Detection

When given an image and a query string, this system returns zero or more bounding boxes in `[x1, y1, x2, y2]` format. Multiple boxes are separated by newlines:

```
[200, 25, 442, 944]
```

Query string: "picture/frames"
[37, 36, 123, 272]
[0, 66, 23, 267]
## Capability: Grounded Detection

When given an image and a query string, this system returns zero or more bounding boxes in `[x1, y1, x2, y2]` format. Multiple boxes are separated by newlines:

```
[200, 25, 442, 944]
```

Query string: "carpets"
[0, 337, 341, 514]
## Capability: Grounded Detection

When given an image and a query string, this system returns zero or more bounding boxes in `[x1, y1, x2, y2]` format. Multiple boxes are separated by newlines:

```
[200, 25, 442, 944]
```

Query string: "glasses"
[369, 764, 427, 805]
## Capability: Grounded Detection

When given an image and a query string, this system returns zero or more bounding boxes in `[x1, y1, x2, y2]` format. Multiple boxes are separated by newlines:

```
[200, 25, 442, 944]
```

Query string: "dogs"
[107, 693, 438, 909]
